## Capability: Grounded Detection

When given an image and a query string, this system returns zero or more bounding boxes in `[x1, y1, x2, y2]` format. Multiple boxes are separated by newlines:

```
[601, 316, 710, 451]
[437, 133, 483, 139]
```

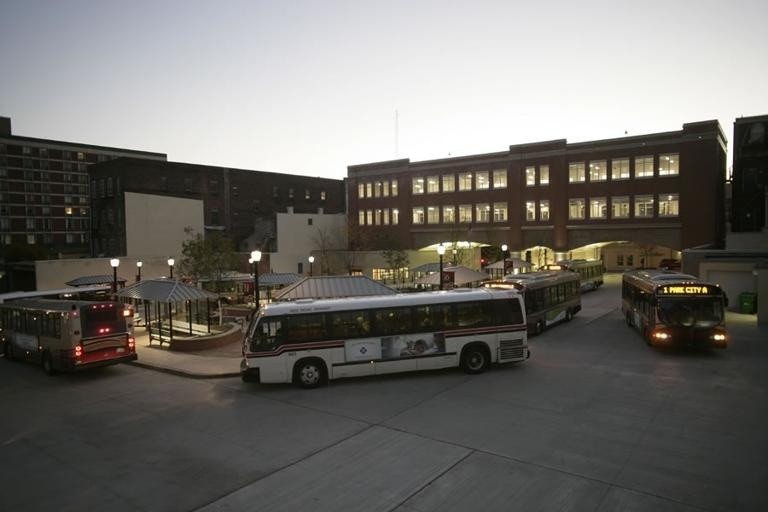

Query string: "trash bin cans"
[739, 291, 757, 314]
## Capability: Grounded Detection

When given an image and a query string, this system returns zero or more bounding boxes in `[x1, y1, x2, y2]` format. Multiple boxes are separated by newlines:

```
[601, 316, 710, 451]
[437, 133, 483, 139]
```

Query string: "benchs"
[158, 316, 211, 337]
[133, 313, 141, 326]
[149, 326, 174, 349]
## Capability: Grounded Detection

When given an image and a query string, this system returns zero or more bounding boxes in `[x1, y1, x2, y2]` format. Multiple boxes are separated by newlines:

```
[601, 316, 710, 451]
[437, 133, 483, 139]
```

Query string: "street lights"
[167, 256, 175, 279]
[137, 259, 143, 305]
[110, 255, 120, 301]
[309, 253, 315, 276]
[437, 241, 446, 290]
[250, 247, 262, 310]
[249, 256, 254, 276]
[501, 241, 508, 277]
[452, 248, 457, 266]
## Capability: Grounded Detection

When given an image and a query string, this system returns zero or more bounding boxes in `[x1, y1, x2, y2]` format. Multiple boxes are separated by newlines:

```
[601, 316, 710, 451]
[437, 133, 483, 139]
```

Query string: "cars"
[659, 259, 681, 270]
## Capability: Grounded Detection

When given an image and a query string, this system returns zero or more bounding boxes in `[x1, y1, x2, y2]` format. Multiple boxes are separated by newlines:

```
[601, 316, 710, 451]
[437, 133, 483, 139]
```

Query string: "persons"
[419, 333, 446, 355]
[401, 339, 429, 355]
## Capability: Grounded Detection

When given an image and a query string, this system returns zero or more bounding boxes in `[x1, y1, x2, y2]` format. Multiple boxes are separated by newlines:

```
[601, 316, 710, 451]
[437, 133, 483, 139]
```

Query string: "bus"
[557, 258, 604, 294]
[478, 270, 582, 336]
[0, 298, 138, 377]
[155, 270, 255, 312]
[621, 267, 728, 349]
[240, 286, 530, 390]
[0, 285, 114, 304]
